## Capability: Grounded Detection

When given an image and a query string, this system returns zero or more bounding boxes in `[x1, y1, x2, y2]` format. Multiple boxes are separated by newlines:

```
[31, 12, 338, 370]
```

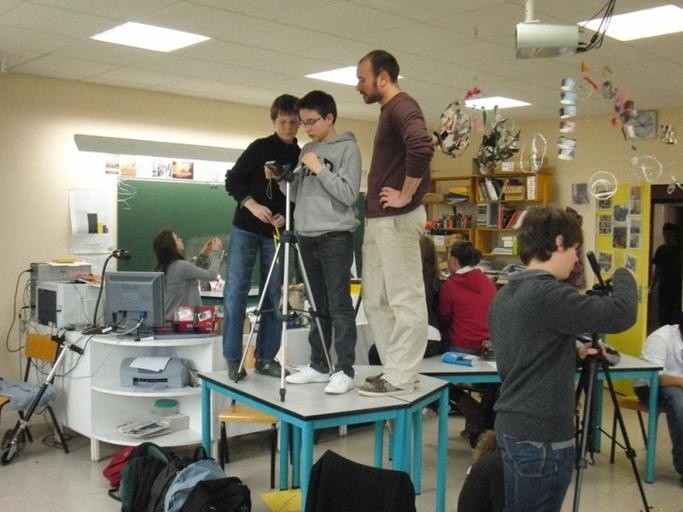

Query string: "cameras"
[265, 156, 321, 183]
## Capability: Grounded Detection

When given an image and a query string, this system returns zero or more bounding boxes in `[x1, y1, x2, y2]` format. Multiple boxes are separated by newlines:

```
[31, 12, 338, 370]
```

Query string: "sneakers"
[228, 357, 419, 397]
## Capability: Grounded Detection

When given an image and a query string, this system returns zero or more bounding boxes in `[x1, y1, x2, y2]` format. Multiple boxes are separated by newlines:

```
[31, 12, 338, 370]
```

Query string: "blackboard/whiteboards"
[117, 177, 367, 292]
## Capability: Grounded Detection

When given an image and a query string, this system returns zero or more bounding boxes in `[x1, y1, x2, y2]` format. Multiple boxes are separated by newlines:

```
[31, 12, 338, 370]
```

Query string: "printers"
[120, 358, 189, 388]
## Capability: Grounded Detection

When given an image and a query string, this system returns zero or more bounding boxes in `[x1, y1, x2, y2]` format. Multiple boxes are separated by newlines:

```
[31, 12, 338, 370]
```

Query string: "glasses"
[299, 116, 322, 126]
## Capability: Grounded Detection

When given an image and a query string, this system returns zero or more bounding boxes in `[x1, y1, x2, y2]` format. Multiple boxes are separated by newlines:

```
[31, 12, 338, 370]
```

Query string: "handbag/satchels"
[103, 443, 251, 512]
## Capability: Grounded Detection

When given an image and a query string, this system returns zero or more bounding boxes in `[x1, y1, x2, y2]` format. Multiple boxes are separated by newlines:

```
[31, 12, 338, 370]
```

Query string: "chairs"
[216, 340, 281, 483]
[0, 333, 70, 463]
[610, 388, 666, 464]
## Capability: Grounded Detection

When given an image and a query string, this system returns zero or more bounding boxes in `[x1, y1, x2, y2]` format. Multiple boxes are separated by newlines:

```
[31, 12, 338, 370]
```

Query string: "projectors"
[515, 24, 579, 59]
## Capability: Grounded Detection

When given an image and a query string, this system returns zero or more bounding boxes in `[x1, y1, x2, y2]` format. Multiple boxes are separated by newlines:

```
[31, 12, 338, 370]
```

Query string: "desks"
[405, 348, 665, 498]
[193, 362, 451, 512]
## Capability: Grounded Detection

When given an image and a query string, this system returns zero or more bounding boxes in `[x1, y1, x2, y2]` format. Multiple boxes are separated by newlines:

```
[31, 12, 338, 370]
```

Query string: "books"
[421, 176, 534, 256]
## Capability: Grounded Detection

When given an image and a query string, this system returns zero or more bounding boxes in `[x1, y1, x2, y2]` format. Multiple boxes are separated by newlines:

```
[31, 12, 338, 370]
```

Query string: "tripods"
[573, 334, 650, 512]
[235, 183, 333, 401]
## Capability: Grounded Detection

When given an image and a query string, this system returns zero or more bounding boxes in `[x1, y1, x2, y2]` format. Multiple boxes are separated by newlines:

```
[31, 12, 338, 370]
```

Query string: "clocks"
[625, 109, 656, 140]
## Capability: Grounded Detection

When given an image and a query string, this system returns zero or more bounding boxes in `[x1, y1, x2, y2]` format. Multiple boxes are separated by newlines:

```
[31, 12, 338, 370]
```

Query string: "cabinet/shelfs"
[87, 335, 214, 455]
[426, 167, 551, 268]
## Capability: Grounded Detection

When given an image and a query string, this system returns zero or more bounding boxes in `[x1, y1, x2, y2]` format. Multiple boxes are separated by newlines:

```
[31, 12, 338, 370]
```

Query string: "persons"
[264, 89, 362, 393]
[456, 428, 506, 511]
[368, 237, 446, 364]
[354, 51, 435, 395]
[152, 231, 222, 321]
[648, 223, 682, 324]
[488, 204, 638, 512]
[223, 96, 300, 381]
[439, 240, 499, 438]
[633, 317, 682, 484]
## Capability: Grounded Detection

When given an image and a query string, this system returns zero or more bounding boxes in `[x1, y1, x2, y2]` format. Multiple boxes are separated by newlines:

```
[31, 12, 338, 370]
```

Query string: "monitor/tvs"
[103, 271, 164, 336]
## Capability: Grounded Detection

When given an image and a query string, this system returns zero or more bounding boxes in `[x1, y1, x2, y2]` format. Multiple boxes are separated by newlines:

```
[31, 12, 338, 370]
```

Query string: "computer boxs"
[30, 262, 91, 322]
